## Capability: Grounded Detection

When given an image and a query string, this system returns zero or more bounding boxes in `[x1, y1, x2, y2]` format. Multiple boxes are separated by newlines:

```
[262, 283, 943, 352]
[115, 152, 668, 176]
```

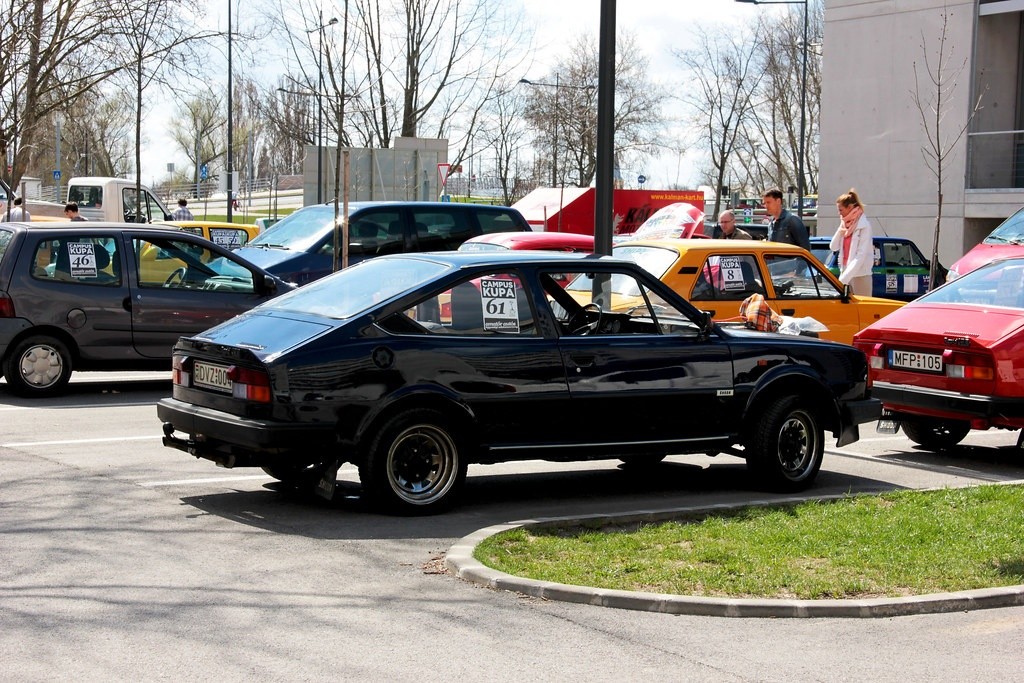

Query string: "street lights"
[734, 0, 808, 217]
[305, 10, 339, 205]
[520, 72, 596, 189]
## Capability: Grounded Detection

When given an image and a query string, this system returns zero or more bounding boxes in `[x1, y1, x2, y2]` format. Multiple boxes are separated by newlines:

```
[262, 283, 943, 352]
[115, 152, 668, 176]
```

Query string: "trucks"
[494, 185, 705, 240]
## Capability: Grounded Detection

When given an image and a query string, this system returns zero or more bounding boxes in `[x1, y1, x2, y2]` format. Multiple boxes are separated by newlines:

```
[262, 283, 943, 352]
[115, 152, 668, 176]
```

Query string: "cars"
[793, 236, 949, 304]
[945, 204, 1024, 283]
[530, 239, 908, 346]
[0, 220, 299, 398]
[42, 219, 261, 287]
[415, 203, 711, 325]
[157, 250, 886, 517]
[67, 177, 175, 226]
[851, 253, 1024, 450]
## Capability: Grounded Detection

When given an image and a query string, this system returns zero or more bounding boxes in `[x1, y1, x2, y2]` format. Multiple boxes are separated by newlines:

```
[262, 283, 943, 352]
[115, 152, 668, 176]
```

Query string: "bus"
[791, 194, 819, 217]
[725, 197, 768, 223]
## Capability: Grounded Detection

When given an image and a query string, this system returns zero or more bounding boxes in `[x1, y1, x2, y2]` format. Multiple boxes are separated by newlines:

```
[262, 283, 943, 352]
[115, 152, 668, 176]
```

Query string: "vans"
[203, 200, 533, 288]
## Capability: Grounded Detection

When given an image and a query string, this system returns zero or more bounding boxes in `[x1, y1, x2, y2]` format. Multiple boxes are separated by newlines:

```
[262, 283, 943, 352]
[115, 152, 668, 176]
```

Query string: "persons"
[64, 202, 88, 221]
[762, 189, 810, 277]
[829, 190, 873, 297]
[718, 211, 752, 240]
[1, 198, 32, 221]
[172, 199, 193, 221]
